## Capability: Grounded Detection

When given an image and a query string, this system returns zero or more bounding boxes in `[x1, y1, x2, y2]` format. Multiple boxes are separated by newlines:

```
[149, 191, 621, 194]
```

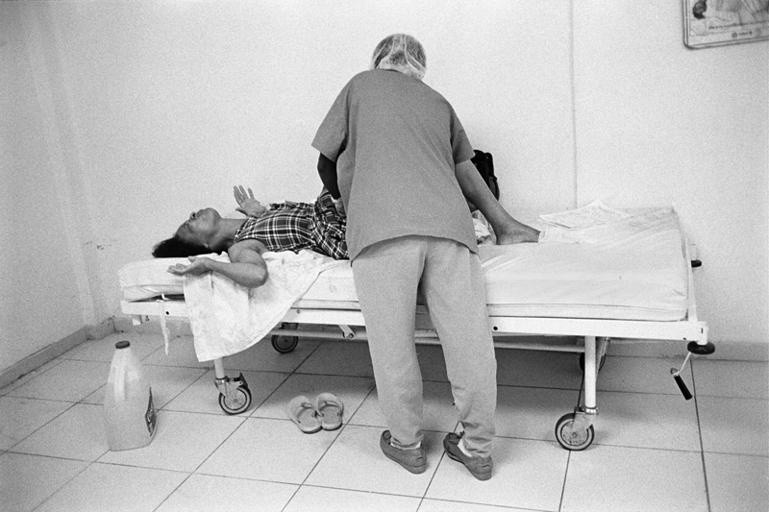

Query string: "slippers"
[317, 392, 343, 430]
[285, 394, 320, 435]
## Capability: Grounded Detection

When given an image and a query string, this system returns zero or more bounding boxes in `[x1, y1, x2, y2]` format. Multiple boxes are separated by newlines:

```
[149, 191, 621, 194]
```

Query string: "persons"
[153, 149, 541, 287]
[310, 33, 499, 482]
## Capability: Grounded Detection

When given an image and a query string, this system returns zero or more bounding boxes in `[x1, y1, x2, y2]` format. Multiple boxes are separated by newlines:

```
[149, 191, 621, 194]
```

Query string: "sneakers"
[443, 431, 492, 482]
[380, 429, 425, 474]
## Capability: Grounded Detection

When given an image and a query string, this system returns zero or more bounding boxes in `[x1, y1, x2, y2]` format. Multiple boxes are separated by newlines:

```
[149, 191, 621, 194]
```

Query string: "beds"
[120, 205, 709, 414]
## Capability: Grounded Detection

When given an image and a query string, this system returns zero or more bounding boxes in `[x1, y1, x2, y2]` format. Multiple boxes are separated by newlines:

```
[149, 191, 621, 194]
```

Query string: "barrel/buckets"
[103, 339, 158, 452]
